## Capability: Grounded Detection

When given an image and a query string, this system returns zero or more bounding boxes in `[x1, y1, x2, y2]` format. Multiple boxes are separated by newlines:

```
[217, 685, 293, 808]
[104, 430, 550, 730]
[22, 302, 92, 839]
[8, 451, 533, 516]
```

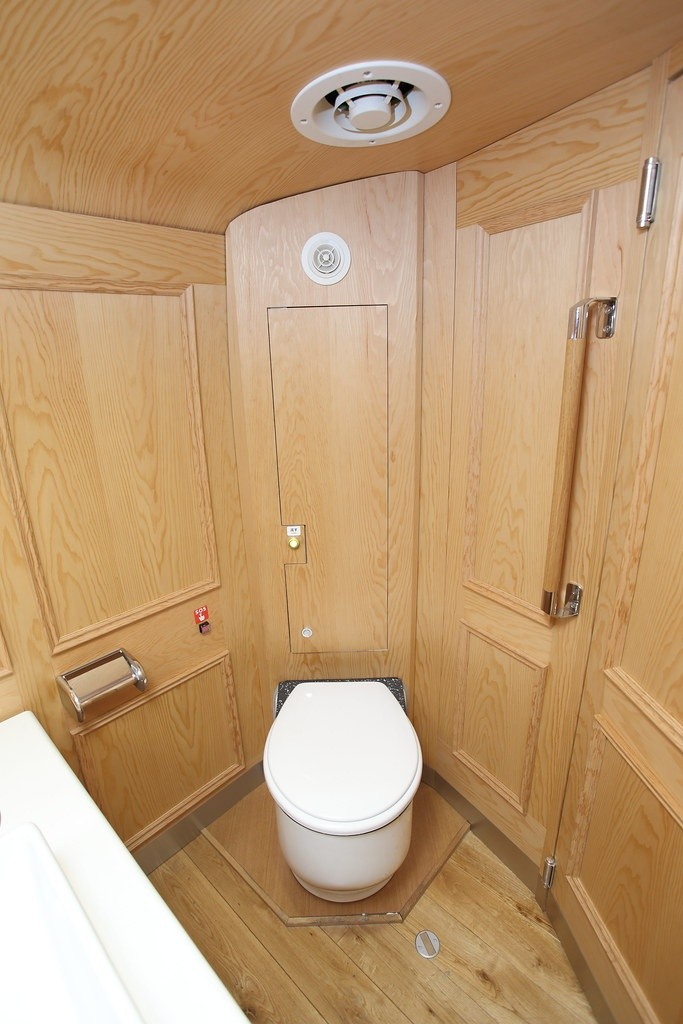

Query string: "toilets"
[262, 682, 423, 904]
